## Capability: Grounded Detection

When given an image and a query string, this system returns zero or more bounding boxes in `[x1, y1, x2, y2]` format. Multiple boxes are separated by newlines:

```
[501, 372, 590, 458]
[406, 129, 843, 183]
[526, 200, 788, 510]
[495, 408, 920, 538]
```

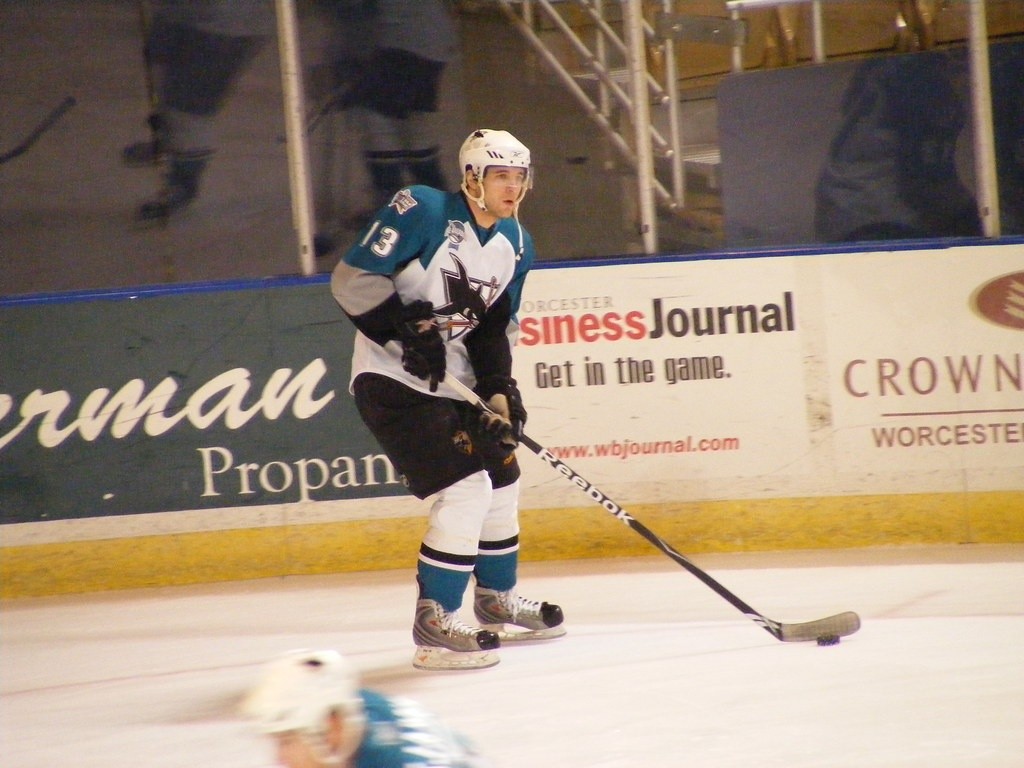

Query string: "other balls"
[817, 635, 840, 646]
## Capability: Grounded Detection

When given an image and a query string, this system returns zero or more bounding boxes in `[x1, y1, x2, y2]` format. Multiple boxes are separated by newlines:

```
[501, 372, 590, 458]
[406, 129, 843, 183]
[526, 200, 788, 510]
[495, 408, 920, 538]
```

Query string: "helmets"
[458, 128, 534, 213]
[231, 649, 363, 740]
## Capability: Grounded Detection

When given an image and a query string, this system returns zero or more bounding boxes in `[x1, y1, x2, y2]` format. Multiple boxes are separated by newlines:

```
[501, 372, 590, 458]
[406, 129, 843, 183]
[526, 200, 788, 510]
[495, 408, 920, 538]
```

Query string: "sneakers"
[125, 131, 168, 162]
[411, 597, 503, 670]
[472, 585, 566, 642]
[134, 172, 198, 224]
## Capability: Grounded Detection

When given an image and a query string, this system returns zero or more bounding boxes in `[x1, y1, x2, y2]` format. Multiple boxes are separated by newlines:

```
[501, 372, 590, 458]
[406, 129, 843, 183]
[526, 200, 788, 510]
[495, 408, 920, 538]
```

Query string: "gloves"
[390, 296, 447, 392]
[468, 376, 525, 450]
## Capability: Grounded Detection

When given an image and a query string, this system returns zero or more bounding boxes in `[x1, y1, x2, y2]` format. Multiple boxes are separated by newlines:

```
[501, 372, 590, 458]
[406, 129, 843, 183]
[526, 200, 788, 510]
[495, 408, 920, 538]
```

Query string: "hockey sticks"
[384, 340, 862, 643]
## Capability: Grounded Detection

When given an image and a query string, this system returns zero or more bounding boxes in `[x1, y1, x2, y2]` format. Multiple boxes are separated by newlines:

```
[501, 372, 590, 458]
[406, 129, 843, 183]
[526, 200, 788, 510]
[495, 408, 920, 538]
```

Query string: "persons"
[124, 0, 457, 259]
[330, 129, 569, 671]
[243, 647, 488, 768]
